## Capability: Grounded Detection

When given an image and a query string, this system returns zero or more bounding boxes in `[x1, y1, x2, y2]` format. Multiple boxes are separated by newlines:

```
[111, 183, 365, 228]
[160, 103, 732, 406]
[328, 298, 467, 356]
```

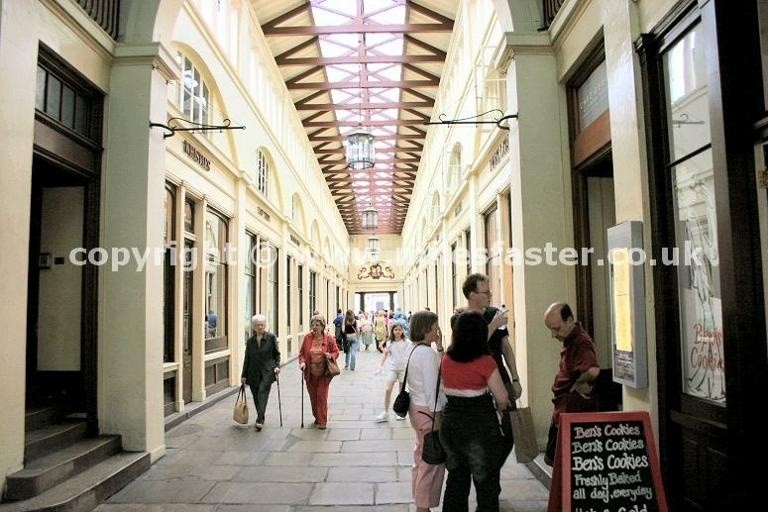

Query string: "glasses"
[478, 290, 491, 294]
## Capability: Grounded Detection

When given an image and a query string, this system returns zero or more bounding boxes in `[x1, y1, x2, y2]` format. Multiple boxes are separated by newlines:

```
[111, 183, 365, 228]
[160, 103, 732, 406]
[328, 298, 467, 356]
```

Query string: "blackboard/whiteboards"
[560, 411, 668, 512]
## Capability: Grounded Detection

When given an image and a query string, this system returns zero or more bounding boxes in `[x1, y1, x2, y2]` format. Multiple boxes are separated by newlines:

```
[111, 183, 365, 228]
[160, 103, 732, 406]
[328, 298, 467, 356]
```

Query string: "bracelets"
[512, 377, 521, 384]
[436, 347, 443, 352]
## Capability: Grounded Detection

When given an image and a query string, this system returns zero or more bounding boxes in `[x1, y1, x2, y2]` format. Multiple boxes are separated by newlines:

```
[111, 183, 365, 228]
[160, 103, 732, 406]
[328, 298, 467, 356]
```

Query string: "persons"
[333, 307, 435, 371]
[239, 314, 281, 431]
[439, 309, 512, 511]
[541, 299, 602, 467]
[313, 310, 319, 316]
[205, 316, 208, 339]
[209, 308, 216, 340]
[374, 325, 415, 424]
[448, 272, 522, 466]
[404, 308, 446, 512]
[298, 313, 341, 429]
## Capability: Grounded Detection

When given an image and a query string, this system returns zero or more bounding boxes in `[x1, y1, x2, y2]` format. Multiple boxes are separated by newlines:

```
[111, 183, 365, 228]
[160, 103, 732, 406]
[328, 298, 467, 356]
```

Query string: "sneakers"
[376, 412, 389, 421]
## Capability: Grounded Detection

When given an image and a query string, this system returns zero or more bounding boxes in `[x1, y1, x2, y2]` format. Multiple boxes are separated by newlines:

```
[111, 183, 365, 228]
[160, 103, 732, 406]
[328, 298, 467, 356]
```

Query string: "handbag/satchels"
[233, 384, 248, 424]
[509, 406, 540, 462]
[325, 360, 340, 376]
[393, 390, 410, 418]
[422, 430, 447, 464]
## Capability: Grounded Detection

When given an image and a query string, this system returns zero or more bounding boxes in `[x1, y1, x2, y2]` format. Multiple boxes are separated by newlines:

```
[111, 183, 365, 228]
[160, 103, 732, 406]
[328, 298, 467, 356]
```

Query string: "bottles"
[497, 303, 507, 330]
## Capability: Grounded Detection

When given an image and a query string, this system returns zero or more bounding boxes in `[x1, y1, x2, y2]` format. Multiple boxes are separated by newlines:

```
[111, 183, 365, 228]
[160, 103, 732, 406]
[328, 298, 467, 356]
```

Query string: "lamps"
[367, 230, 380, 254]
[345, 39, 376, 171]
[361, 200, 379, 230]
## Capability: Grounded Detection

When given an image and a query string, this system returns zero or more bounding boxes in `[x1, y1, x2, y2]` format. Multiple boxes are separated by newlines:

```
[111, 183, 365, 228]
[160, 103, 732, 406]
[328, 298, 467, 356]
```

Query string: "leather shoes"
[255, 422, 263, 429]
